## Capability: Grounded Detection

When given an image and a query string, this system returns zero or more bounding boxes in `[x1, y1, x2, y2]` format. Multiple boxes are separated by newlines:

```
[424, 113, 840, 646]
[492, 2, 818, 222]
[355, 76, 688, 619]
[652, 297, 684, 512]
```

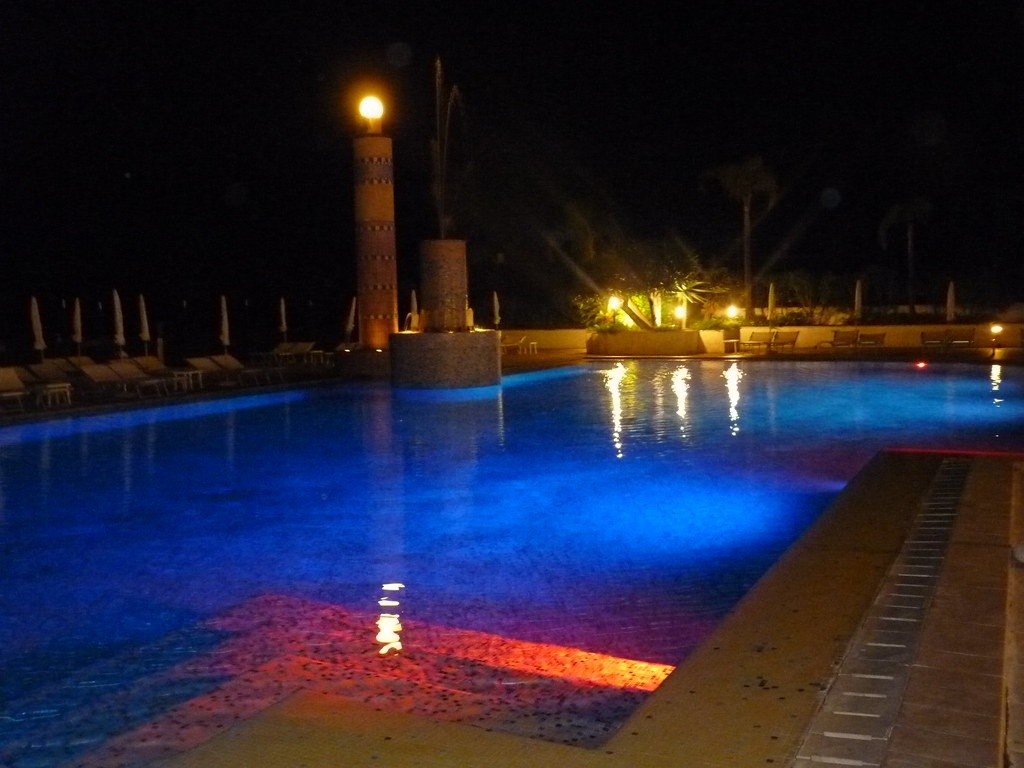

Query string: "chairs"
[108, 359, 170, 400]
[28, 361, 79, 397]
[280, 342, 315, 365]
[184, 356, 243, 389]
[828, 330, 860, 348]
[0, 368, 28, 410]
[500, 334, 527, 355]
[79, 364, 142, 400]
[946, 328, 976, 345]
[44, 357, 81, 379]
[854, 333, 886, 347]
[209, 354, 288, 386]
[68, 355, 111, 391]
[764, 331, 799, 354]
[921, 331, 946, 345]
[134, 355, 174, 377]
[268, 343, 293, 358]
[740, 331, 775, 354]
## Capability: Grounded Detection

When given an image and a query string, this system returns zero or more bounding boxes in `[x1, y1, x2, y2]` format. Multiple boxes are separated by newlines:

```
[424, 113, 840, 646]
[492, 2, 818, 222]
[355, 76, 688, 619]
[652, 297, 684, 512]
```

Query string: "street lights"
[351, 96, 399, 349]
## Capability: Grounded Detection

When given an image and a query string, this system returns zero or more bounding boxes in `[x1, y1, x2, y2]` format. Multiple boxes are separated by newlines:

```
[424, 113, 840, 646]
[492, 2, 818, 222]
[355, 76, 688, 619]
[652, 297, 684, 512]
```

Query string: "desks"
[44, 383, 72, 408]
[308, 349, 325, 363]
[173, 369, 205, 391]
[723, 340, 740, 355]
[518, 339, 537, 355]
